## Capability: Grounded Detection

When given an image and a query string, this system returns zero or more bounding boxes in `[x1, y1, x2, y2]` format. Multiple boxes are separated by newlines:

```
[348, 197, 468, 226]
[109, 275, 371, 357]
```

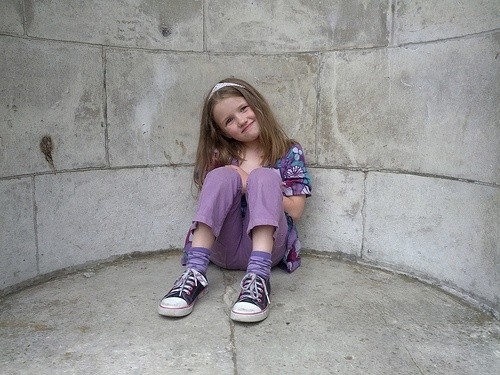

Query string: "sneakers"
[230, 273, 272, 324]
[158, 268, 209, 318]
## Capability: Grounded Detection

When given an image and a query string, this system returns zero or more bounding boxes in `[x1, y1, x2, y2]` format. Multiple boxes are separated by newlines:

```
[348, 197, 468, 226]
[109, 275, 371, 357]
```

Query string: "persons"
[158, 78, 312, 322]
